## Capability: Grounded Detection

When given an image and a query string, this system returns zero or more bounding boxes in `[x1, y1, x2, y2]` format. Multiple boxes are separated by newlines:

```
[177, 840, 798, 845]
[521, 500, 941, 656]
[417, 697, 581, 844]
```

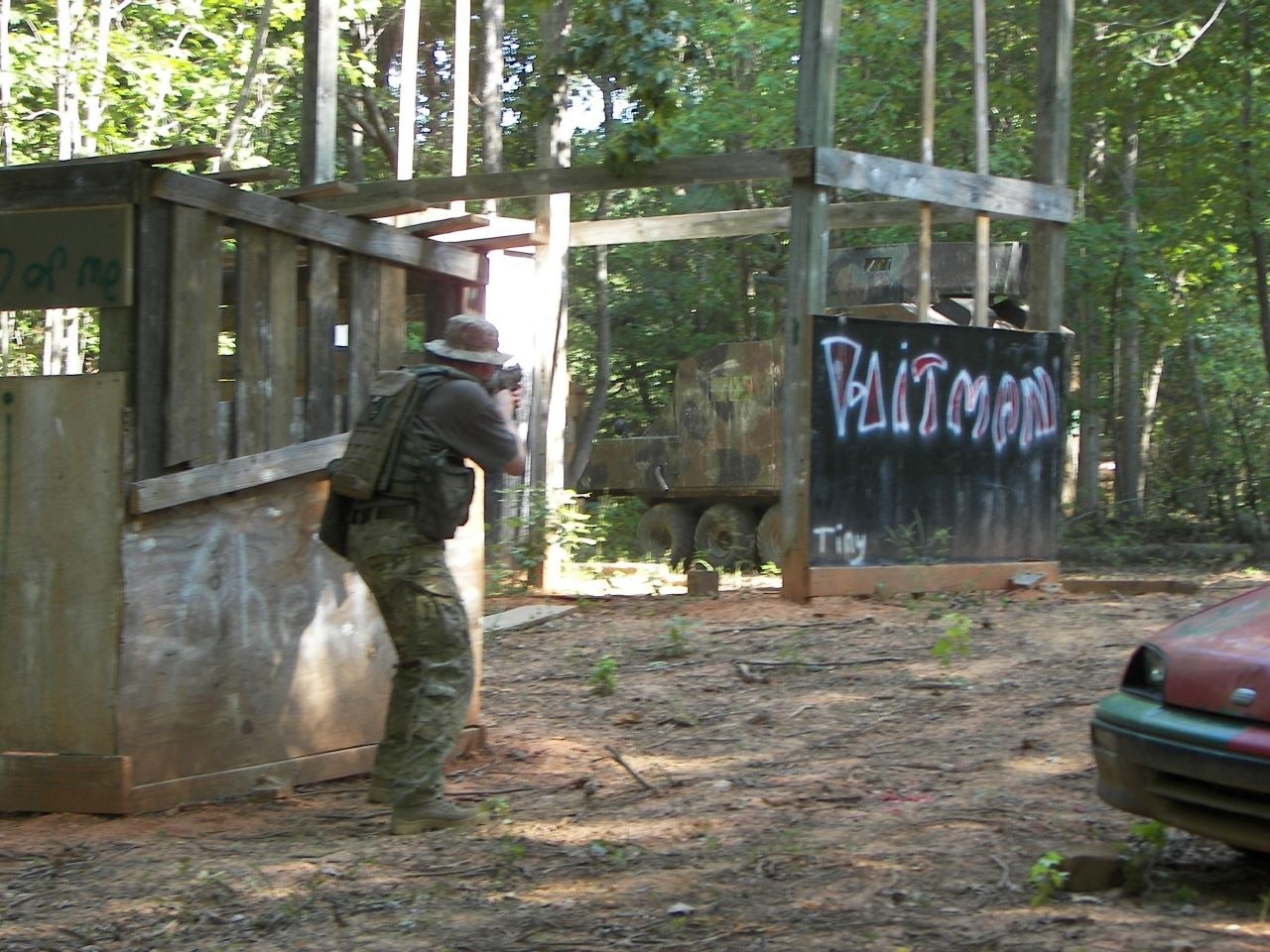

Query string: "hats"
[423, 313, 513, 364]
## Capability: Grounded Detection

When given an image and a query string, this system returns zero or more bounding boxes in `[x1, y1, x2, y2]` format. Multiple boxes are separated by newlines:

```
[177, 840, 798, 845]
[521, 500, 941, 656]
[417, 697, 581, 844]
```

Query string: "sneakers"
[387, 798, 490, 835]
[369, 781, 392, 811]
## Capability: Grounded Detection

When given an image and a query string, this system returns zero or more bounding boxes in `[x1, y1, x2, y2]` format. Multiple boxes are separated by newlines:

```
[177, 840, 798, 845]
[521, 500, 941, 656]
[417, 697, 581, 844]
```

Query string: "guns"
[480, 363, 525, 424]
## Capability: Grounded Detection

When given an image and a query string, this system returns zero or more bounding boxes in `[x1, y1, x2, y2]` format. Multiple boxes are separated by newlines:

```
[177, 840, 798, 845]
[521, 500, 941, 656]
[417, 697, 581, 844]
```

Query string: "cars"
[1086, 581, 1269, 866]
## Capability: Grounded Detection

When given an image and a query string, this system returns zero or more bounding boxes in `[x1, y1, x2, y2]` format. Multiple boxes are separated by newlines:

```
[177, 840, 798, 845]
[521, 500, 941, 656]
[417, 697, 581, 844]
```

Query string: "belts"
[349, 503, 407, 525]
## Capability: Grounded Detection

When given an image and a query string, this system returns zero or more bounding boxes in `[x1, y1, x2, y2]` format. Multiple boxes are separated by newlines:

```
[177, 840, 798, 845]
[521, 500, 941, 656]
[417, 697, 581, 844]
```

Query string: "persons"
[348, 314, 523, 836]
